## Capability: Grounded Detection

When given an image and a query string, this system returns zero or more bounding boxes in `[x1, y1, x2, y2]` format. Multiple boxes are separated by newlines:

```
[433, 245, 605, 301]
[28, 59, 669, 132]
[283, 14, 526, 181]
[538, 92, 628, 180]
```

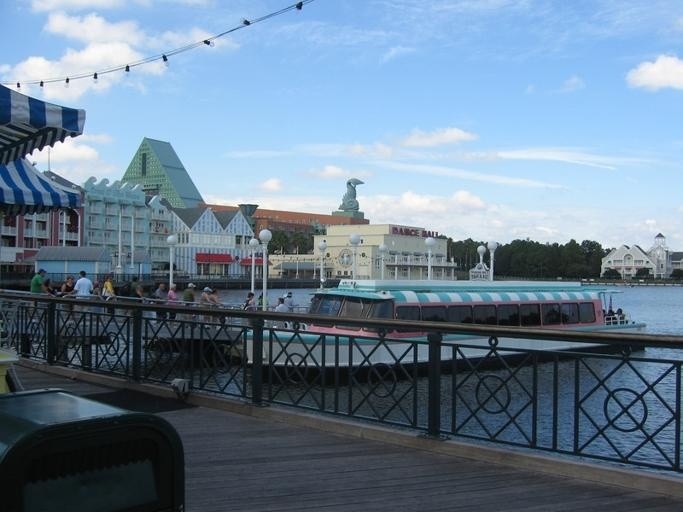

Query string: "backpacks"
[240, 298, 252, 310]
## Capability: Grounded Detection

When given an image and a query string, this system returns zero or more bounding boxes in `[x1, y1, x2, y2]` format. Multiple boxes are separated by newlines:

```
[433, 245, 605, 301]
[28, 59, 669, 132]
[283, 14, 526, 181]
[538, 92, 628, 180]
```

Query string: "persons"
[30, 268, 220, 328]
[244, 292, 296, 313]
[603, 306, 627, 326]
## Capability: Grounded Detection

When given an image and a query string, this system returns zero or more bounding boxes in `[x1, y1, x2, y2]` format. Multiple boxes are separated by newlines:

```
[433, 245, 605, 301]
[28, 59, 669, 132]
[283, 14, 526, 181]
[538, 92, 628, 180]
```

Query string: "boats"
[238, 277, 647, 383]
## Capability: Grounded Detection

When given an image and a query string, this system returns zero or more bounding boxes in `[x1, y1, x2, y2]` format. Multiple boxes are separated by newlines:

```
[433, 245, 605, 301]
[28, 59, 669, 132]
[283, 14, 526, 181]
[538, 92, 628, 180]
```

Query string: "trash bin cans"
[0, 388, 186, 512]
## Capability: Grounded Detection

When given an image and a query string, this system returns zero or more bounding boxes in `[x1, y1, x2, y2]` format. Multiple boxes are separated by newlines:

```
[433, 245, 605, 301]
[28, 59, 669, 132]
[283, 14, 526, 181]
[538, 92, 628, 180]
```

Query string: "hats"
[37, 268, 46, 274]
[188, 283, 197, 287]
[203, 287, 213, 292]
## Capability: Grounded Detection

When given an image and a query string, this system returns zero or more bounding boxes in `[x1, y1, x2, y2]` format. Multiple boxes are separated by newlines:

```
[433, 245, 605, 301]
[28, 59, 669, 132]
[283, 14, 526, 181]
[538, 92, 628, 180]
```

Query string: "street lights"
[476, 244, 487, 265]
[423, 237, 435, 281]
[248, 238, 260, 294]
[378, 242, 387, 281]
[316, 239, 328, 290]
[165, 235, 177, 289]
[349, 233, 360, 283]
[486, 239, 498, 282]
[258, 228, 272, 311]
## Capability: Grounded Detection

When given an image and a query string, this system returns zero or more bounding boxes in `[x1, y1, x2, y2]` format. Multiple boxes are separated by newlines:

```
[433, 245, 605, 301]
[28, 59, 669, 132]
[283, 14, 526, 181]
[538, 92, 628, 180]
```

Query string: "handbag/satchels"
[102, 288, 113, 297]
